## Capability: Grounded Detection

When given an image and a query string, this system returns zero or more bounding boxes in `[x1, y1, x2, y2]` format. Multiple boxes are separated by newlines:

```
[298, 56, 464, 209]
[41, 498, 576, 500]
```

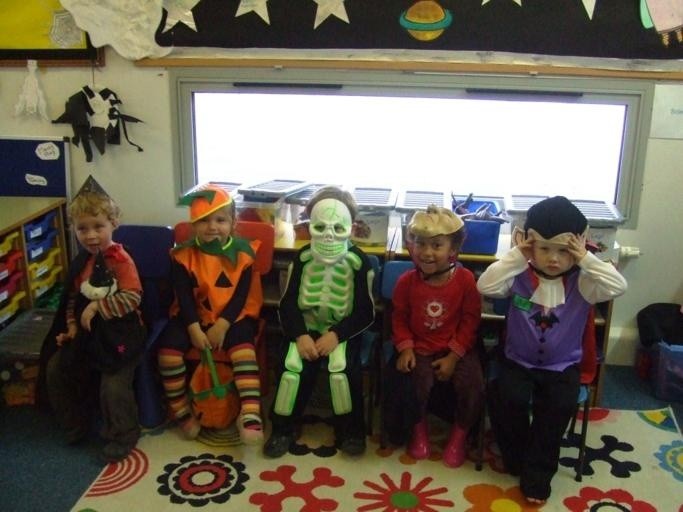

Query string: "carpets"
[56, 408, 681, 511]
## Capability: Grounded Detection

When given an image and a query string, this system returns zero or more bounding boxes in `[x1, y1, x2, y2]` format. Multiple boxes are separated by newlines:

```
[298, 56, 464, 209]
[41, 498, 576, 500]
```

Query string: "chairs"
[28, 224, 172, 425]
[496, 300, 619, 482]
[272, 257, 382, 437]
[376, 255, 484, 471]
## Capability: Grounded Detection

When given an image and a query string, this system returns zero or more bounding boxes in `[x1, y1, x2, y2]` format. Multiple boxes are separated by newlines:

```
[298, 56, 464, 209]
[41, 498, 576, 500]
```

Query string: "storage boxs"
[238, 171, 303, 221]
[506, 189, 546, 247]
[633, 309, 682, 403]
[184, 175, 240, 207]
[351, 187, 395, 246]
[287, 181, 340, 227]
[450, 189, 503, 253]
[395, 183, 444, 248]
[572, 194, 623, 261]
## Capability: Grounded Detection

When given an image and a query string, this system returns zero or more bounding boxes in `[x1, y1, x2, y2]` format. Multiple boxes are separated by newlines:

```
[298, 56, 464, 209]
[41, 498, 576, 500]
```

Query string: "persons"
[154, 182, 265, 445]
[389, 204, 483, 467]
[39, 173, 151, 466]
[263, 184, 377, 457]
[473, 197, 628, 504]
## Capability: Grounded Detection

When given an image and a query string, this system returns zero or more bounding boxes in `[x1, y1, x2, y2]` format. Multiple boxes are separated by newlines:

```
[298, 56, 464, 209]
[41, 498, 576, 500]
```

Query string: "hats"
[525, 197, 589, 248]
[191, 185, 229, 224]
[72, 175, 109, 199]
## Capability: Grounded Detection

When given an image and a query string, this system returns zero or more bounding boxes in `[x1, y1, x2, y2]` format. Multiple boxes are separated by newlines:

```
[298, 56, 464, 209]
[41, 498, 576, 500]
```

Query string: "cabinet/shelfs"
[1, 197, 68, 326]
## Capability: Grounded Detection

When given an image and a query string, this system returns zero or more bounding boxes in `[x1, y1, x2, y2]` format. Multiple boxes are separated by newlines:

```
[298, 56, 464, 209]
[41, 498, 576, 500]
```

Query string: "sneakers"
[99, 436, 134, 463]
[177, 410, 201, 440]
[264, 433, 300, 457]
[236, 413, 265, 446]
[407, 419, 432, 459]
[340, 428, 366, 454]
[443, 424, 469, 467]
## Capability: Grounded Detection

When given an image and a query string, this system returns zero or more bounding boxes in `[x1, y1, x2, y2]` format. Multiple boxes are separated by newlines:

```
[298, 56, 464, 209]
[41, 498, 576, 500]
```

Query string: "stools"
[183, 318, 276, 421]
